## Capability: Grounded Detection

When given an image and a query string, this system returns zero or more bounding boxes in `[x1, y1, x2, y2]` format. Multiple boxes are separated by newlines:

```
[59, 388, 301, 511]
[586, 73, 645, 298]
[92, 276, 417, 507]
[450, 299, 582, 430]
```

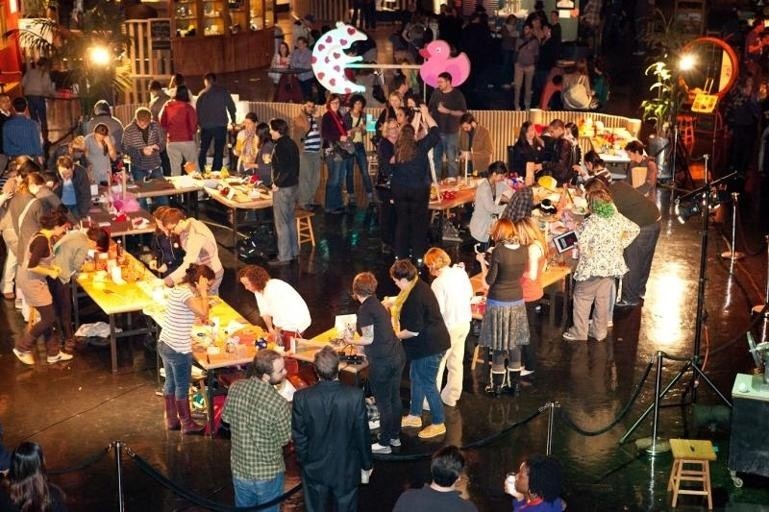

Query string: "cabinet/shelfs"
[168, 0, 276, 76]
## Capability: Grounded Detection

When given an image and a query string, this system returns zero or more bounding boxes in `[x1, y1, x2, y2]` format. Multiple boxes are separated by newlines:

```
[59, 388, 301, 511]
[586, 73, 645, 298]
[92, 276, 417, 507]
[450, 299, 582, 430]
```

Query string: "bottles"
[751, 368, 763, 390]
[117, 239, 123, 256]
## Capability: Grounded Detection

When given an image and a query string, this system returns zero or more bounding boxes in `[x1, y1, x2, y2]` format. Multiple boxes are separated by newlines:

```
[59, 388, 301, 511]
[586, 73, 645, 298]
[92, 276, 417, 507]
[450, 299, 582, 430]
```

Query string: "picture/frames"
[555, 0, 575, 10]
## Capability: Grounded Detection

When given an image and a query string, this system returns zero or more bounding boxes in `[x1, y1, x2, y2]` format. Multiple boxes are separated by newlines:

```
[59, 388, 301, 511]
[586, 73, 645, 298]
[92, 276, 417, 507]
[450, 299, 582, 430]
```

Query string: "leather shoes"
[561, 331, 589, 342]
[266, 260, 291, 268]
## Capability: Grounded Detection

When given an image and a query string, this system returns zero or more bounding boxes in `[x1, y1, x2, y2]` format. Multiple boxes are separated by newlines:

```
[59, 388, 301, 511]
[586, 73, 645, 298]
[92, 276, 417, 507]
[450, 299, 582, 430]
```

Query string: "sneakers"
[389, 438, 402, 447]
[401, 414, 422, 428]
[12, 347, 37, 366]
[417, 421, 447, 439]
[370, 442, 392, 454]
[46, 350, 75, 364]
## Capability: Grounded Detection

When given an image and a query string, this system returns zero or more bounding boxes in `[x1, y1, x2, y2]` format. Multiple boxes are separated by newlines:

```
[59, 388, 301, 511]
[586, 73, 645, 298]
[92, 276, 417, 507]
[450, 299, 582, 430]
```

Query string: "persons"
[0, 172, 47, 299]
[233, 112, 259, 176]
[121, 108, 171, 215]
[377, 119, 400, 253]
[3, 97, 46, 169]
[458, 112, 494, 178]
[158, 264, 215, 433]
[344, 273, 407, 454]
[41, 136, 93, 176]
[504, 454, 566, 511]
[47, 228, 109, 348]
[150, 206, 186, 279]
[586, 180, 617, 327]
[396, 107, 411, 126]
[392, 445, 478, 511]
[220, 349, 292, 512]
[580, 1, 646, 87]
[563, 60, 596, 112]
[159, 85, 202, 177]
[390, 104, 440, 254]
[322, 93, 356, 214]
[0, 155, 34, 209]
[0, 441, 68, 512]
[636, 115, 670, 221]
[13, 217, 74, 365]
[244, 122, 275, 234]
[563, 190, 641, 341]
[407, 95, 438, 184]
[85, 124, 118, 187]
[429, 72, 466, 180]
[236, 266, 310, 375]
[592, 175, 661, 306]
[261, 118, 300, 265]
[381, 260, 451, 439]
[4, 159, 40, 194]
[424, 248, 473, 411]
[344, 92, 377, 207]
[531, 118, 576, 187]
[565, 122, 581, 186]
[22, 57, 54, 144]
[48, 156, 92, 221]
[293, 97, 323, 211]
[294, 15, 385, 99]
[476, 218, 530, 398]
[393, 77, 408, 107]
[165, 74, 197, 111]
[0, 93, 13, 155]
[743, 18, 769, 103]
[487, 176, 563, 251]
[15, 172, 59, 310]
[85, 101, 125, 177]
[147, 80, 170, 122]
[292, 37, 314, 104]
[388, 4, 561, 88]
[625, 141, 656, 204]
[469, 161, 508, 278]
[573, 149, 612, 188]
[509, 121, 545, 182]
[162, 209, 223, 298]
[514, 218, 548, 376]
[292, 345, 374, 512]
[374, 92, 407, 131]
[515, 24, 538, 111]
[197, 73, 237, 172]
[269, 42, 292, 92]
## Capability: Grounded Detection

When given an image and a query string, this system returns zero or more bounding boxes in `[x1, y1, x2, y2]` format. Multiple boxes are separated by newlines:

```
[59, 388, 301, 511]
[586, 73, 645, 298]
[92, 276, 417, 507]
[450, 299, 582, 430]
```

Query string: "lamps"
[674, 202, 702, 224]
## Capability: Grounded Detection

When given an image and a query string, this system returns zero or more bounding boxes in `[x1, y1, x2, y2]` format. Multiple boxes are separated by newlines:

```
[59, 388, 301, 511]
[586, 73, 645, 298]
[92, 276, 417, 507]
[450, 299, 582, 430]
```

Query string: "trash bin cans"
[86, 335, 112, 365]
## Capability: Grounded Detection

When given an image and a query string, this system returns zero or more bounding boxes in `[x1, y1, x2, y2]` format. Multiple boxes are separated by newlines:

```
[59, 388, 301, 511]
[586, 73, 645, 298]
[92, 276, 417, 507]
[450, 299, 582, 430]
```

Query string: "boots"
[164, 393, 182, 431]
[174, 399, 205, 434]
[502, 368, 522, 393]
[484, 368, 507, 395]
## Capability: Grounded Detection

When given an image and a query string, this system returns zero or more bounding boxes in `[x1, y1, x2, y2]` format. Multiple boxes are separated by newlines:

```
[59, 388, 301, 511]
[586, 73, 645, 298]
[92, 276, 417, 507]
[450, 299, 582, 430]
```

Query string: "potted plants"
[1, 0, 134, 136]
[639, 62, 675, 179]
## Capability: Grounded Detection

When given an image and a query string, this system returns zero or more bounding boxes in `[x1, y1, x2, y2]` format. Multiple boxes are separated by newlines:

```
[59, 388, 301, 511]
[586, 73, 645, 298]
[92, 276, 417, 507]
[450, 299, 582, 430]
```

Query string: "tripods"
[619, 151, 733, 445]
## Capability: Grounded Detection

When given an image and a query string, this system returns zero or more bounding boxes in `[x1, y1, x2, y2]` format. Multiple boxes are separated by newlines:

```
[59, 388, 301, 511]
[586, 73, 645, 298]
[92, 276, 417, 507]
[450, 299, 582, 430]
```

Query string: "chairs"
[1, 70, 22, 100]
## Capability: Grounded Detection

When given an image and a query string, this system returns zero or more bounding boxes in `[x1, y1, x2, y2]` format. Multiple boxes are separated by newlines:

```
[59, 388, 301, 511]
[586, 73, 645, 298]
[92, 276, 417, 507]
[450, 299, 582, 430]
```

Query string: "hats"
[537, 175, 560, 193]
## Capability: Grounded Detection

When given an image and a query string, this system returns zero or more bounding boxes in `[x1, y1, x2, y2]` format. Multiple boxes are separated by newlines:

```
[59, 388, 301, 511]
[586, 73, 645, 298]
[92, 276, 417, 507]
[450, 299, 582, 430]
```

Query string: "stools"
[295, 210, 315, 249]
[677, 116, 694, 144]
[667, 438, 716, 510]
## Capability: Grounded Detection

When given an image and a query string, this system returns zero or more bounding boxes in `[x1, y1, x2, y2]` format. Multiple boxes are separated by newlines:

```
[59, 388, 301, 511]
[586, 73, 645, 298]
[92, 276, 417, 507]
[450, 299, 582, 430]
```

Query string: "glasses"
[168, 220, 179, 232]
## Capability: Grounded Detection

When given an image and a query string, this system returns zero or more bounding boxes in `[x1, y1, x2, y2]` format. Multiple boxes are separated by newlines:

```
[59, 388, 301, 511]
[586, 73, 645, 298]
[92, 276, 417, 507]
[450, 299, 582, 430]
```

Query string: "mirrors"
[674, 37, 738, 98]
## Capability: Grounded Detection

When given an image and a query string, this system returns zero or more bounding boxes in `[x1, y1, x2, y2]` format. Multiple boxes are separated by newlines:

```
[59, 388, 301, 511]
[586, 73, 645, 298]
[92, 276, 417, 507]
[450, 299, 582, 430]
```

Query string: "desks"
[143, 295, 284, 440]
[428, 184, 476, 229]
[271, 68, 312, 104]
[69, 249, 173, 375]
[90, 176, 204, 220]
[285, 327, 401, 387]
[85, 208, 156, 250]
[589, 128, 648, 179]
[469, 267, 571, 372]
[187, 171, 274, 248]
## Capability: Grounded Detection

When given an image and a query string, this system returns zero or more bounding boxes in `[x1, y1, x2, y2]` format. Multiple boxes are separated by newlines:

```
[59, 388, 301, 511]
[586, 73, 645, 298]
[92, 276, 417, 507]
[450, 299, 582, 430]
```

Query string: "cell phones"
[654, 54, 693, 201]
